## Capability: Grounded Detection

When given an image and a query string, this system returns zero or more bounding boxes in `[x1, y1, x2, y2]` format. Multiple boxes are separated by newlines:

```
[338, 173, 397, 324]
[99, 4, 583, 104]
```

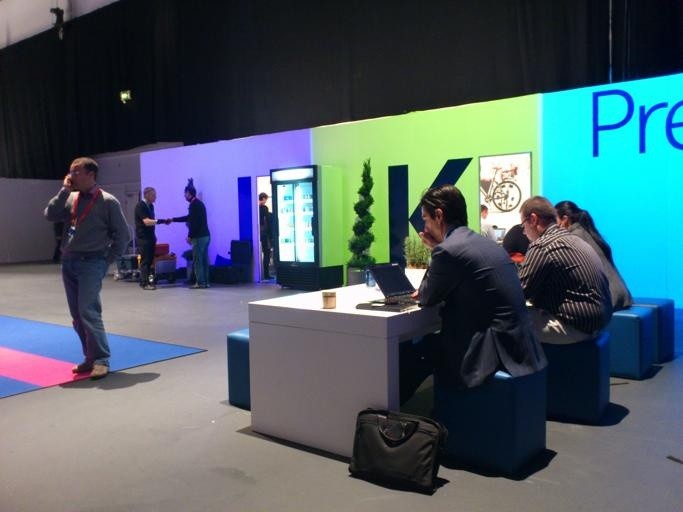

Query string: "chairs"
[209, 240, 251, 284]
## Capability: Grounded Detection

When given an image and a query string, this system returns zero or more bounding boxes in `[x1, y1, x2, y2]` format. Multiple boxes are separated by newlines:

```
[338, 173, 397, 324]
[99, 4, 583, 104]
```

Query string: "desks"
[249, 269, 442, 460]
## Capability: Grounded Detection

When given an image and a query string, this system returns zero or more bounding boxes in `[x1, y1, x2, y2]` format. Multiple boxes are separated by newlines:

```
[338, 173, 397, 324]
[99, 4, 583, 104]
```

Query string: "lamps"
[50, 0, 65, 39]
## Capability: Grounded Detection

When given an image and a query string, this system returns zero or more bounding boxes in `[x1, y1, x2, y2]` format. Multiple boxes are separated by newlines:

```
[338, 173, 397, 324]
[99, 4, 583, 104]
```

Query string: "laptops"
[493, 227, 507, 241]
[368, 262, 419, 305]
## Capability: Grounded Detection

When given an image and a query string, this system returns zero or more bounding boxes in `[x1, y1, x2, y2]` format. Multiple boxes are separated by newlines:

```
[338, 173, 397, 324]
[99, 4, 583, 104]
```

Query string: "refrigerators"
[269, 165, 345, 290]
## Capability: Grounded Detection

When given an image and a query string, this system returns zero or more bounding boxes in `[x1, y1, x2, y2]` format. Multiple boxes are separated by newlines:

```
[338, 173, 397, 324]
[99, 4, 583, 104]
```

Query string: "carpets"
[0, 315, 208, 398]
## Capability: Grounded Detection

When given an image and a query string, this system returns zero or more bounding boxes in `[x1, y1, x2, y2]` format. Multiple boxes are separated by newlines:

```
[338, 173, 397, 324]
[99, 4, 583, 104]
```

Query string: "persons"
[258, 190, 277, 281]
[554, 201, 634, 309]
[42, 157, 130, 378]
[408, 184, 549, 390]
[168, 182, 211, 289]
[480, 204, 496, 244]
[517, 195, 614, 346]
[51, 221, 65, 264]
[134, 186, 167, 291]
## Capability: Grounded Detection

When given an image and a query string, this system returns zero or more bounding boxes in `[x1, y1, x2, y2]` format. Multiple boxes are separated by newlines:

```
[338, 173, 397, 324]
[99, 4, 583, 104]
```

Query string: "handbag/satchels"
[348, 407, 449, 497]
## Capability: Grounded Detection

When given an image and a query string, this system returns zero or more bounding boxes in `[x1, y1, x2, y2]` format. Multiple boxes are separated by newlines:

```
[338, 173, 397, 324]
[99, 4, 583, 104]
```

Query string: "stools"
[433, 363, 550, 475]
[543, 330, 611, 422]
[631, 297, 674, 363]
[226, 327, 250, 409]
[607, 307, 657, 379]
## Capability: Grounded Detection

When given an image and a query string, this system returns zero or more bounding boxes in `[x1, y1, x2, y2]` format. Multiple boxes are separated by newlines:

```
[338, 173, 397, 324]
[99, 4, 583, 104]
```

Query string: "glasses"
[519, 218, 528, 229]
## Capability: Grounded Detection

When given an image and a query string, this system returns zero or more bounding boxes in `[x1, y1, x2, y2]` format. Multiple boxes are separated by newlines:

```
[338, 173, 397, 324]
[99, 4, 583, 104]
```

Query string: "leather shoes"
[72, 362, 94, 373]
[90, 364, 109, 379]
[190, 282, 206, 288]
[143, 282, 156, 289]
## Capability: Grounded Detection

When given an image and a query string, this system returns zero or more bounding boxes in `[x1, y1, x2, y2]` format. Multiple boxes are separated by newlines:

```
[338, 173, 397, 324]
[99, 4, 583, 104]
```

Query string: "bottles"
[321, 292, 337, 309]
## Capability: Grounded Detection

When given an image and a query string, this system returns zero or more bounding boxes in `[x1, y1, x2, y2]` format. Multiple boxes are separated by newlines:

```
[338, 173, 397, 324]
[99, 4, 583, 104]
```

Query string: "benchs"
[277, 261, 343, 289]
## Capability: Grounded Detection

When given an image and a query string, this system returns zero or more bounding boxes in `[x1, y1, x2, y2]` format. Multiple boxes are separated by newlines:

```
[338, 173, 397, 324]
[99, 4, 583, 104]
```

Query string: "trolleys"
[114, 226, 176, 285]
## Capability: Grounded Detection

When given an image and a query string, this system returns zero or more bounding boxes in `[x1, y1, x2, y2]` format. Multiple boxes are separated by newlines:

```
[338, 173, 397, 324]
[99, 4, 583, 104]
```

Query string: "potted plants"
[405, 235, 430, 268]
[345, 158, 373, 285]
